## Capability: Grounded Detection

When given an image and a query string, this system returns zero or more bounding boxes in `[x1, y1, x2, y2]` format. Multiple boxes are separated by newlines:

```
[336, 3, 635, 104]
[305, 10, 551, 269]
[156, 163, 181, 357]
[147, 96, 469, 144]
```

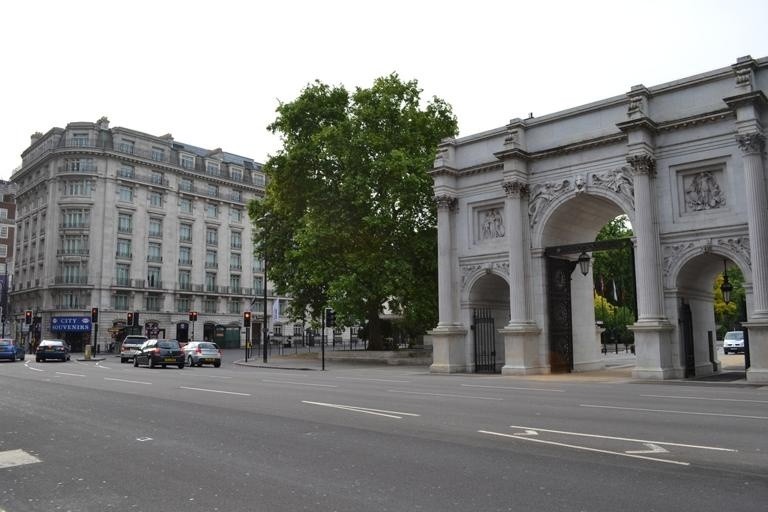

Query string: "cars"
[0, 339, 26, 362]
[134, 339, 185, 369]
[182, 342, 221, 368]
[35, 339, 71, 362]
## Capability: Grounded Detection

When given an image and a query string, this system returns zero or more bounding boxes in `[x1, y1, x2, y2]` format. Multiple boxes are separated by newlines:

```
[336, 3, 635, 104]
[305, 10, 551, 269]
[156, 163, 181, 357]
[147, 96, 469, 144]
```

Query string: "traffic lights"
[326, 308, 337, 327]
[189, 312, 192, 320]
[244, 312, 251, 327]
[91, 308, 98, 323]
[128, 313, 133, 325]
[193, 312, 197, 321]
[25, 310, 33, 324]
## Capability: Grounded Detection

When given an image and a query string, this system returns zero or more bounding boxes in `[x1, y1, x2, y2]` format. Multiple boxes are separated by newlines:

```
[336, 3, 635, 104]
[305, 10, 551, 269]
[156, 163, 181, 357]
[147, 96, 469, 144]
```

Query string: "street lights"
[257, 212, 271, 364]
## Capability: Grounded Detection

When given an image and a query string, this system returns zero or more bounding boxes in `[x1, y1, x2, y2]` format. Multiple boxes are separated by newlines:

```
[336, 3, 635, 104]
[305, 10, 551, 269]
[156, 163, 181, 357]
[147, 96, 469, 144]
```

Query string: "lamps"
[721, 258, 733, 305]
[564, 250, 591, 277]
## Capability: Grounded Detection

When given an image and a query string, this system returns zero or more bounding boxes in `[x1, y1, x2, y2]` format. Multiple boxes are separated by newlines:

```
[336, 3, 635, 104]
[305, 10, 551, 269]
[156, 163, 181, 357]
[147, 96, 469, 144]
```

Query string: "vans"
[120, 334, 149, 363]
[723, 331, 745, 354]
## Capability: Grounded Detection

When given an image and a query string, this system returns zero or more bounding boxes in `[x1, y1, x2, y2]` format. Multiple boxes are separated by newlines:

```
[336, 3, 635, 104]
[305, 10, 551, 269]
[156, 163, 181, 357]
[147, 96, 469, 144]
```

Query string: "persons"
[718, 238, 751, 266]
[595, 170, 635, 210]
[481, 209, 504, 237]
[690, 172, 721, 206]
[663, 241, 693, 276]
[529, 181, 551, 227]
